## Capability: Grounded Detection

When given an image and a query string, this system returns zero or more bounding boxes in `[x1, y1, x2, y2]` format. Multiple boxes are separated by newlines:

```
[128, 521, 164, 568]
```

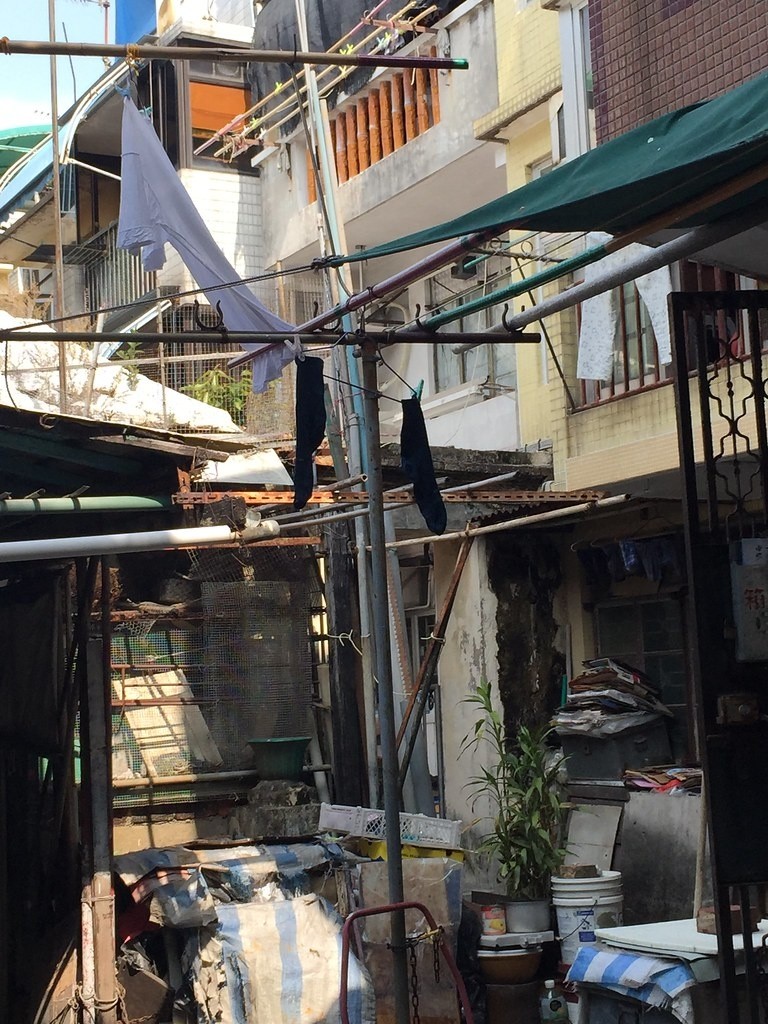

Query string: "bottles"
[540, 981, 568, 1024]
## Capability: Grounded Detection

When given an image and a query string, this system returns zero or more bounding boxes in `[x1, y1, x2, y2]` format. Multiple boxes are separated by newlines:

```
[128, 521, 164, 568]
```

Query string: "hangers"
[570, 501, 679, 553]
[292, 292, 418, 403]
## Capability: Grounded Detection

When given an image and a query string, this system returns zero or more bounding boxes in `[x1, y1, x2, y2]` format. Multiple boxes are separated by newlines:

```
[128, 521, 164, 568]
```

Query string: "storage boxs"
[462, 895, 506, 935]
[317, 801, 462, 851]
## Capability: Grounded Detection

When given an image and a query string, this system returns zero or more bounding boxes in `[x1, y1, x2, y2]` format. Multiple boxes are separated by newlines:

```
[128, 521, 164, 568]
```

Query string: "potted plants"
[454, 674, 602, 935]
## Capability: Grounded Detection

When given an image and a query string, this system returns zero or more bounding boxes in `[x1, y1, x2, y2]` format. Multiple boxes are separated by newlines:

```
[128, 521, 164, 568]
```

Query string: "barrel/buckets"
[553, 871, 626, 970]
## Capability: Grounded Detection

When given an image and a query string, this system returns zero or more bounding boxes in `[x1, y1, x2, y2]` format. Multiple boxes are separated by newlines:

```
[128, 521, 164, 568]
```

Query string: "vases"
[247, 735, 313, 782]
